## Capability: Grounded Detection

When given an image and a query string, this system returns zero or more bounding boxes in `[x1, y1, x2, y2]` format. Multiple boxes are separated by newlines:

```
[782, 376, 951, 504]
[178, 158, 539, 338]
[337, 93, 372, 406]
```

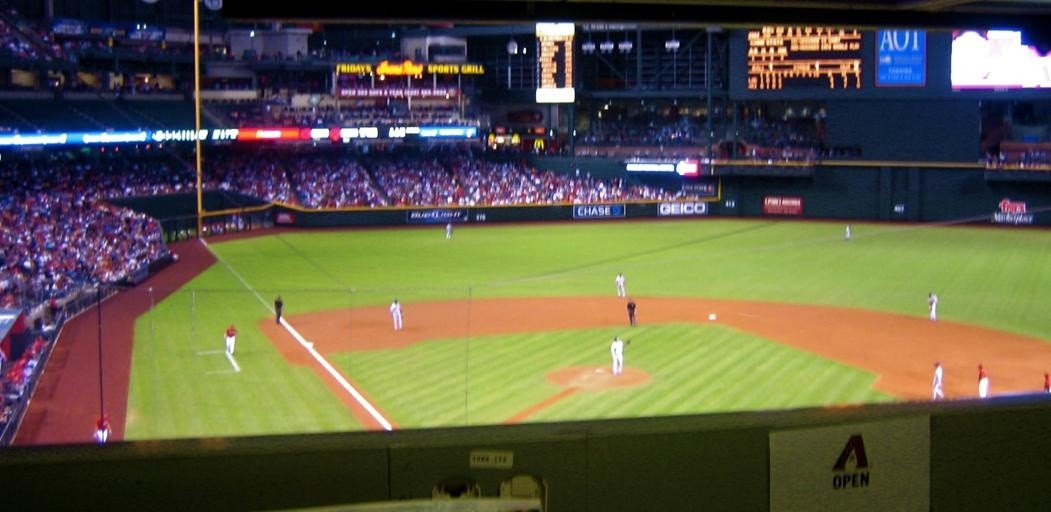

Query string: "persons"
[1043, 373, 1051, 393]
[931, 360, 944, 400]
[982, 147, 1048, 169]
[845, 224, 852, 240]
[1, 7, 832, 446]
[976, 364, 989, 398]
[928, 292, 938, 320]
